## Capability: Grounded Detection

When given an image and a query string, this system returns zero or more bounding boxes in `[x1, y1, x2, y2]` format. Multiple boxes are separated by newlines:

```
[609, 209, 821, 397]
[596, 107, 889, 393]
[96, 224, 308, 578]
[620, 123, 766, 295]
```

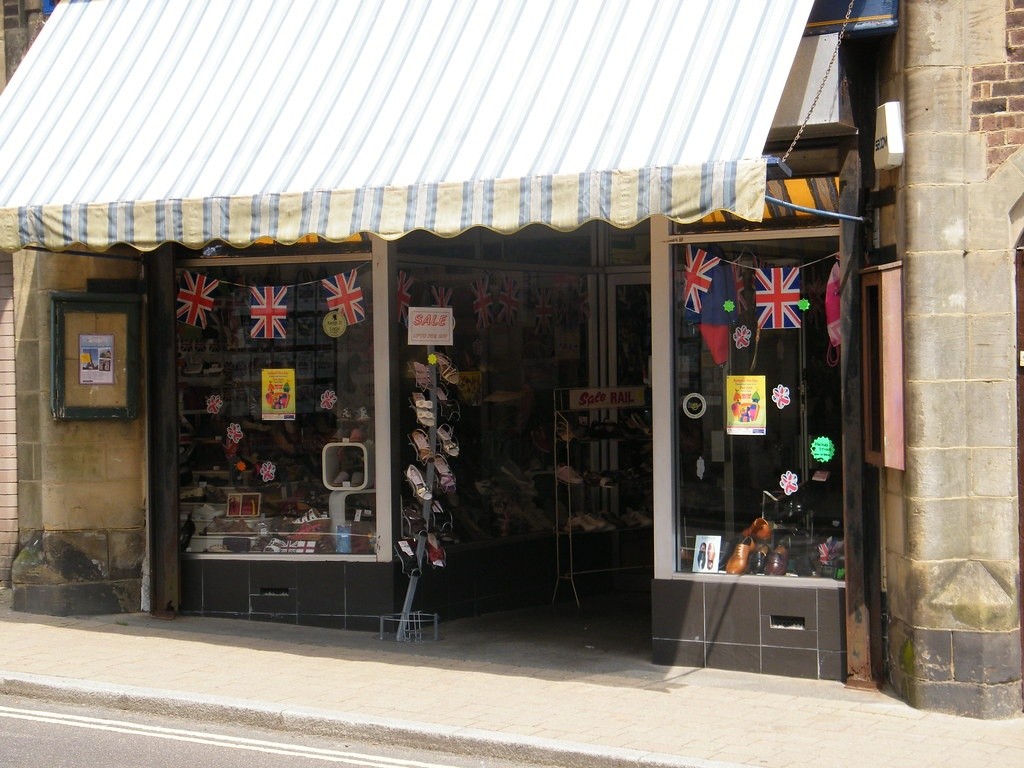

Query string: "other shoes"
[406, 464, 432, 500]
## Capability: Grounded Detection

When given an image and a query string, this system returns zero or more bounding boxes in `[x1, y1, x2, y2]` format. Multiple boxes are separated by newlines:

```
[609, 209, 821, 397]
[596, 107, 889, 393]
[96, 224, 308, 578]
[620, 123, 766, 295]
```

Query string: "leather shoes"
[707, 543, 716, 569]
[698, 542, 706, 570]
[725, 515, 845, 578]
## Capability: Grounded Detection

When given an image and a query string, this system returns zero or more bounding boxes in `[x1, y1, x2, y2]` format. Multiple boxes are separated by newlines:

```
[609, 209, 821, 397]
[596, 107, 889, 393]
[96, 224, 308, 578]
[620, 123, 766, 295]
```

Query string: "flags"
[322, 268, 365, 326]
[250, 285, 287, 339]
[681, 245, 721, 313]
[755, 267, 802, 329]
[394, 270, 587, 333]
[176, 271, 220, 329]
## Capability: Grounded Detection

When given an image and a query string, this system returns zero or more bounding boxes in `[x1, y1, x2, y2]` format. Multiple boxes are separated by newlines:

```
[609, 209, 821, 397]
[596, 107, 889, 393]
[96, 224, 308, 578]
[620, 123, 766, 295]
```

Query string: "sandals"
[405, 503, 427, 537]
[435, 423, 459, 455]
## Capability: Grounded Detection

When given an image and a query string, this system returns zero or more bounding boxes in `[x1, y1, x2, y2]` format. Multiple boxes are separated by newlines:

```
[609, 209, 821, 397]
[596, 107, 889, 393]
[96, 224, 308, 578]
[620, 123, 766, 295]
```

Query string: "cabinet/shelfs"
[178, 438, 376, 554]
[553, 386, 652, 609]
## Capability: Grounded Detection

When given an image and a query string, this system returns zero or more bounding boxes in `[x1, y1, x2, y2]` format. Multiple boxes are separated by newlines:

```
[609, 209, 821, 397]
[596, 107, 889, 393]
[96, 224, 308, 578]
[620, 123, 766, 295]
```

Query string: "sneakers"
[448, 345, 653, 543]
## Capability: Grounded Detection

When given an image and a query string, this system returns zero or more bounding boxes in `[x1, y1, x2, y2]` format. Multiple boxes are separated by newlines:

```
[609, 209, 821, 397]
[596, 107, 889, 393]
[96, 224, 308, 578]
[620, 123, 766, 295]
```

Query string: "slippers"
[435, 383, 460, 422]
[432, 500, 452, 535]
[434, 351, 458, 384]
[395, 543, 420, 577]
[412, 430, 435, 464]
[177, 323, 377, 555]
[413, 361, 434, 390]
[435, 453, 456, 493]
[429, 533, 444, 566]
[413, 392, 435, 427]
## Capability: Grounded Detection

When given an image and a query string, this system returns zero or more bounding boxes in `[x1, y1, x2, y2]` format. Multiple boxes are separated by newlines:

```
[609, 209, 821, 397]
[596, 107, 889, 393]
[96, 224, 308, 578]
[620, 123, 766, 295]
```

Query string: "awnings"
[0, 0, 854, 250]
